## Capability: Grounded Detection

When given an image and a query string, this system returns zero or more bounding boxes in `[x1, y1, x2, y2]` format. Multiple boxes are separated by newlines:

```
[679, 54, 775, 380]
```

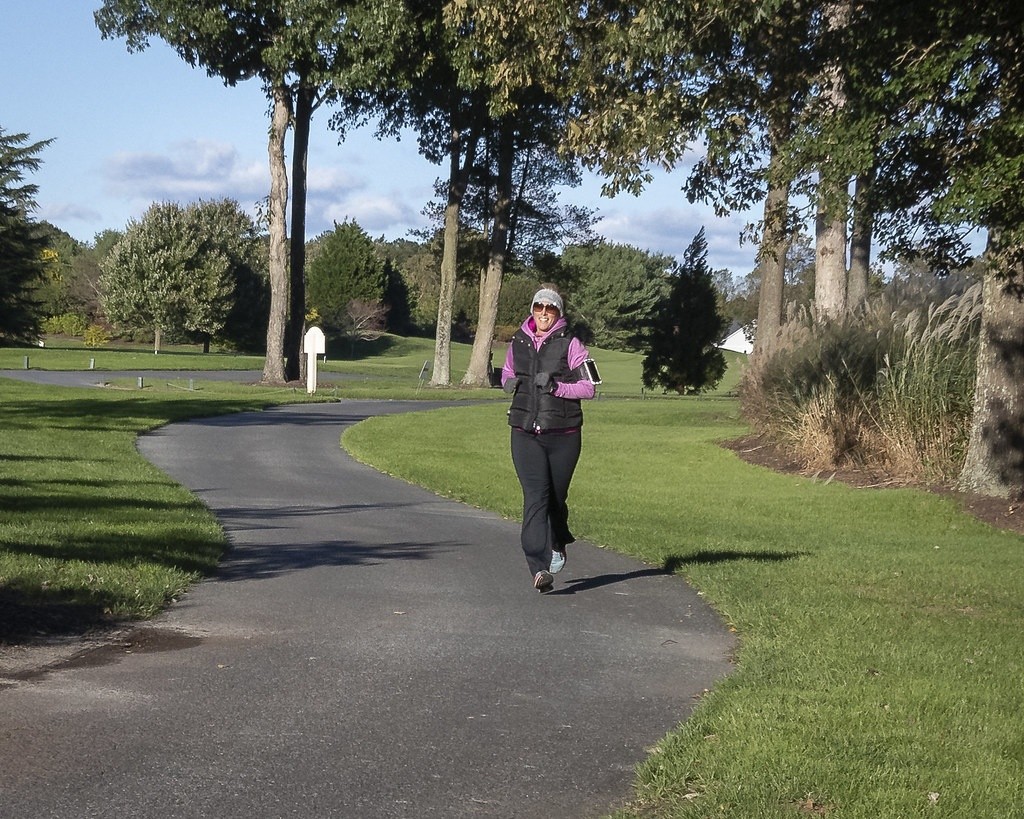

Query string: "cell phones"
[584, 358, 602, 385]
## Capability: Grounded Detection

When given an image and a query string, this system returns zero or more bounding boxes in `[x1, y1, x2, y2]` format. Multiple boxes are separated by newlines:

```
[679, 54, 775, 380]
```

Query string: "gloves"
[533, 372, 559, 395]
[503, 378, 520, 394]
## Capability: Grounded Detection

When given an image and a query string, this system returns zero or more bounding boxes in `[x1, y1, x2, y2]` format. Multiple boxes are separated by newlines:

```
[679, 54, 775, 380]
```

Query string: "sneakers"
[550, 550, 567, 573]
[533, 570, 554, 594]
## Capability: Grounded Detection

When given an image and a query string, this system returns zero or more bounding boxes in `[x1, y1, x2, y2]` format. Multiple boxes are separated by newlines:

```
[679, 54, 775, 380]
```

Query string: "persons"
[500, 288, 603, 596]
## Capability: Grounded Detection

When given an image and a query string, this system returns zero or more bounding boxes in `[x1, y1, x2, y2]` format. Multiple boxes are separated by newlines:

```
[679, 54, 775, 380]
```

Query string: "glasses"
[533, 302, 559, 316]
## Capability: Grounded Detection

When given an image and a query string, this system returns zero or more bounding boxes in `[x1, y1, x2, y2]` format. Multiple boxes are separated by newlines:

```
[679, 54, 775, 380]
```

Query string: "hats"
[530, 289, 564, 317]
